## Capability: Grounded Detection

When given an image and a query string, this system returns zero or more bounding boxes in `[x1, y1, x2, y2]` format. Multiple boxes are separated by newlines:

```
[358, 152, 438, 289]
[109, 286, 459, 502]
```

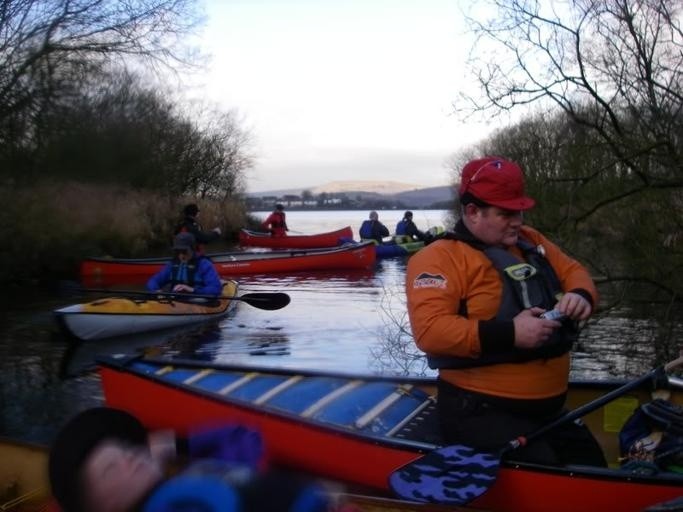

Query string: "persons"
[359, 211, 389, 244]
[145, 231, 223, 298]
[46, 406, 363, 512]
[396, 211, 425, 241]
[261, 204, 289, 251]
[175, 203, 222, 258]
[406, 157, 607, 470]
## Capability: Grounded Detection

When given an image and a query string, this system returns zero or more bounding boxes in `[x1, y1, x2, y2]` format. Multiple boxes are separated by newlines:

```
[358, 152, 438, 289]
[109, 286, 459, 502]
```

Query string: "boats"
[79, 240, 377, 291]
[0, 352, 683, 512]
[238, 226, 354, 248]
[335, 225, 449, 259]
[52, 278, 239, 342]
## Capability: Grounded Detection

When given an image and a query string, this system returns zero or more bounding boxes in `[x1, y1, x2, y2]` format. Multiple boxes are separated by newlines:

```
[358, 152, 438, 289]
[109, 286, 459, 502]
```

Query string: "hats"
[460, 157, 536, 210]
[49, 406, 147, 512]
[173, 232, 196, 249]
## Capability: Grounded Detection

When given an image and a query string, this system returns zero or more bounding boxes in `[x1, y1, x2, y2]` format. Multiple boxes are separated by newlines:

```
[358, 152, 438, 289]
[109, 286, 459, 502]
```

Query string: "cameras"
[540, 307, 567, 323]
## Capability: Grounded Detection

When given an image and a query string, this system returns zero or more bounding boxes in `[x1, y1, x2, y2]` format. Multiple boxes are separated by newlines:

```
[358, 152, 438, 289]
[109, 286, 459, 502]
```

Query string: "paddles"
[240, 229, 271, 236]
[13, 284, 290, 309]
[264, 247, 342, 254]
[389, 357, 683, 503]
